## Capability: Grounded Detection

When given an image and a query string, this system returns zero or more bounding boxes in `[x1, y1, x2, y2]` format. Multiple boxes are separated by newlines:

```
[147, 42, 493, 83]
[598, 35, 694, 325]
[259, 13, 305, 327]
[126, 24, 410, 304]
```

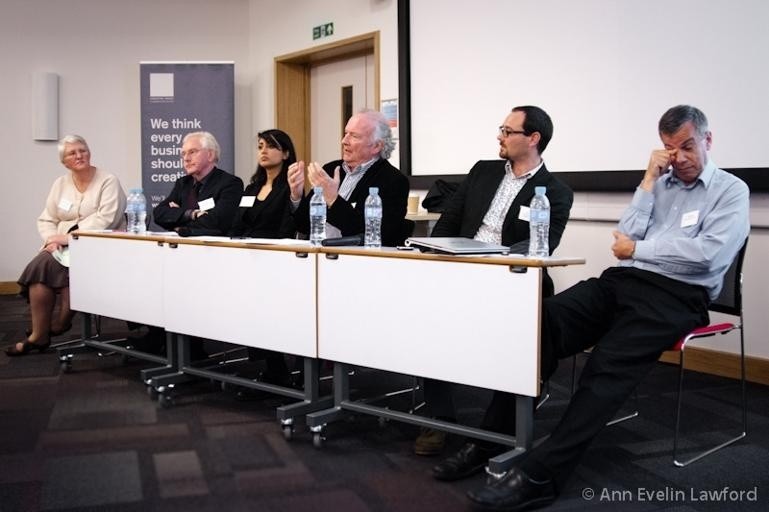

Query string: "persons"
[151, 129, 245, 241]
[405, 103, 577, 456]
[4, 133, 129, 359]
[286, 108, 413, 245]
[233, 127, 307, 239]
[433, 106, 752, 511]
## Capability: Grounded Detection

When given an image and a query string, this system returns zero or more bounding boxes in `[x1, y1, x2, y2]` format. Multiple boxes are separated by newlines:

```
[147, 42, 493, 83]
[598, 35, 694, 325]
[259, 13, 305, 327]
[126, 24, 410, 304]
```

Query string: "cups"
[407, 196, 420, 214]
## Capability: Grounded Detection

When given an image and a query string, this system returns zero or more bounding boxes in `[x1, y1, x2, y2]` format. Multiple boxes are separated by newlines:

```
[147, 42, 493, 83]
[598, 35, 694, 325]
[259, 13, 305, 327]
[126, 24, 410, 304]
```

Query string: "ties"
[185, 183, 198, 210]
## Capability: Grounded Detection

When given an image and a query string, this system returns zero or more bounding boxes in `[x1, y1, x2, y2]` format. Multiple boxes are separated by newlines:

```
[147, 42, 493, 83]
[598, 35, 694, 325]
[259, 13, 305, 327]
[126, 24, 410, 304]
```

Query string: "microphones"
[321, 233, 365, 246]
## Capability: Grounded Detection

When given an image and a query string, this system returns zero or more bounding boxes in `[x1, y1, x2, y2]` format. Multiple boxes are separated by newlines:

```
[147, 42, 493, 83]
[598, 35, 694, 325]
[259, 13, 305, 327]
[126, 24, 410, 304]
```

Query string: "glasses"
[499, 125, 525, 138]
[180, 149, 201, 158]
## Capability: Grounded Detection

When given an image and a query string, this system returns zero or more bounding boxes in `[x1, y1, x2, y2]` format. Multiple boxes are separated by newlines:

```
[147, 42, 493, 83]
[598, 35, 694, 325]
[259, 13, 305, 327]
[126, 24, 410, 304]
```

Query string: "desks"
[309, 245, 588, 485]
[151, 233, 358, 440]
[54, 228, 246, 401]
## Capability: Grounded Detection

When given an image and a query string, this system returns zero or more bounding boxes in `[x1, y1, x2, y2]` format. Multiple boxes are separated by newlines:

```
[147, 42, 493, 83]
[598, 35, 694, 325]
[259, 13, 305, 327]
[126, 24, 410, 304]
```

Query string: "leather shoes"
[235, 379, 272, 401]
[465, 466, 566, 512]
[415, 428, 452, 458]
[426, 439, 504, 484]
[262, 386, 306, 409]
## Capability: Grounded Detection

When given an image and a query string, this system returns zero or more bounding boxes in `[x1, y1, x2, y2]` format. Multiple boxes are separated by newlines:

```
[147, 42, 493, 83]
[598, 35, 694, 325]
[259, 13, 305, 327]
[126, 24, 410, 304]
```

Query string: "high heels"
[4, 339, 50, 354]
[25, 323, 72, 338]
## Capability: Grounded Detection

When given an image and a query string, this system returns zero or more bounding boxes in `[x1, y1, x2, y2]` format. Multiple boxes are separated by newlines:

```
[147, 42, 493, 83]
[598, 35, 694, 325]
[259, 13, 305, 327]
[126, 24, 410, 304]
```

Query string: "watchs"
[629, 241, 636, 259]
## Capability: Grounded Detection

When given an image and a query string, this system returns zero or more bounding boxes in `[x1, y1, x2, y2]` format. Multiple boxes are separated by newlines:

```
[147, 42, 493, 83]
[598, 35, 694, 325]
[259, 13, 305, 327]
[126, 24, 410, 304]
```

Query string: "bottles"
[132, 189, 146, 233]
[528, 186, 549, 257]
[364, 187, 381, 248]
[126, 188, 137, 232]
[310, 186, 326, 246]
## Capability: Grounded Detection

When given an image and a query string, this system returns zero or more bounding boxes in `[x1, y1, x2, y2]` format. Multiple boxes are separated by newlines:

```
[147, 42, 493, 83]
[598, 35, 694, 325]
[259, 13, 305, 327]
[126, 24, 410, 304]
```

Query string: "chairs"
[571, 234, 750, 466]
